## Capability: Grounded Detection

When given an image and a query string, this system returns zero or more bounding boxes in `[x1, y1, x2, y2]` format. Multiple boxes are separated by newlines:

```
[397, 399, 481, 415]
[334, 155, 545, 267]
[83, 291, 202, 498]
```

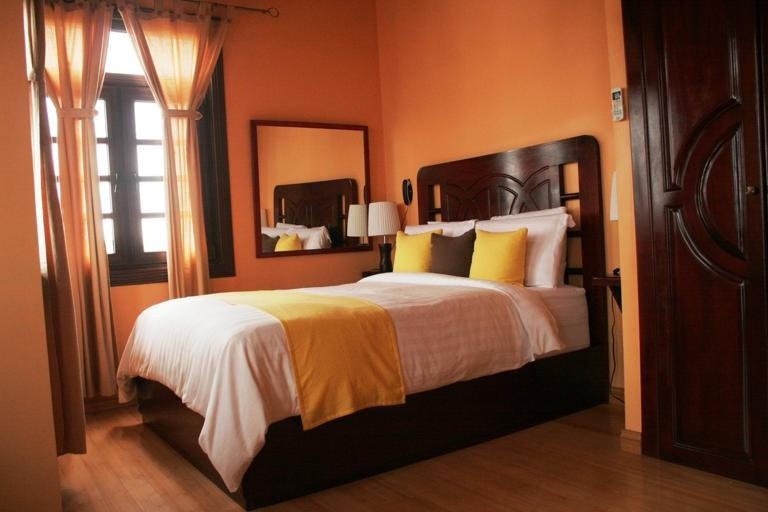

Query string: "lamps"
[345, 202, 368, 247]
[366, 198, 402, 274]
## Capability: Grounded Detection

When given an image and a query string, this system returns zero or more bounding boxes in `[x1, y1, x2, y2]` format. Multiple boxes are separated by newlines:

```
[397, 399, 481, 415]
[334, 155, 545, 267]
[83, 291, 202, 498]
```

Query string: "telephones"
[403, 179, 412, 205]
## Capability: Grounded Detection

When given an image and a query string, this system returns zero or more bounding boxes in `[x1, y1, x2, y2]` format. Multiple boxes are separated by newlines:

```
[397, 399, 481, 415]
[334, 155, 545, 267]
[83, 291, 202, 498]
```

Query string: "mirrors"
[247, 117, 372, 260]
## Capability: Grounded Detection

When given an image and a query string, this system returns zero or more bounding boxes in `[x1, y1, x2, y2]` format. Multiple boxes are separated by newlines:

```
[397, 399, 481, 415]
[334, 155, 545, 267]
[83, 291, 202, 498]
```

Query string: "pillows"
[260, 220, 336, 255]
[389, 205, 584, 292]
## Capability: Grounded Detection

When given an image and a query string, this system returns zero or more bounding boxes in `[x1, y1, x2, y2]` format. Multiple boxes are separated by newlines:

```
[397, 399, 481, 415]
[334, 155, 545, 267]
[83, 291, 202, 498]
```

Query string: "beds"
[259, 176, 366, 252]
[113, 133, 609, 512]
